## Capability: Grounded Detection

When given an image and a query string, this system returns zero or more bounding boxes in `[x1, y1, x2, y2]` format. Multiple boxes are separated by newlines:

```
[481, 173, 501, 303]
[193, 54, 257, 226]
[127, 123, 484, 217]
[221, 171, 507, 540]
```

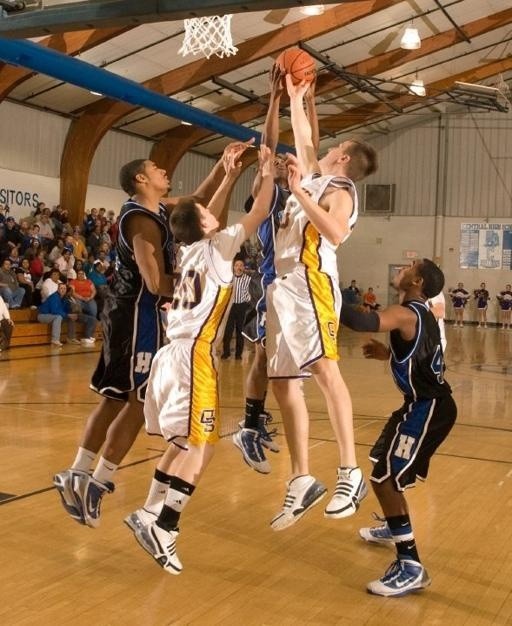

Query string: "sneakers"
[229, 409, 282, 477]
[365, 552, 433, 599]
[322, 463, 371, 521]
[269, 473, 330, 532]
[50, 466, 116, 530]
[358, 519, 400, 546]
[51, 336, 97, 347]
[121, 503, 184, 576]
[453, 323, 511, 331]
[221, 352, 243, 361]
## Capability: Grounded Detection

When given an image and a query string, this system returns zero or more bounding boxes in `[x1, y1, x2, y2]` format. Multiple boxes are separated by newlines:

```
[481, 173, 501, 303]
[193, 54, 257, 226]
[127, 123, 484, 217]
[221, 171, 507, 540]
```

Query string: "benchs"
[7, 309, 107, 348]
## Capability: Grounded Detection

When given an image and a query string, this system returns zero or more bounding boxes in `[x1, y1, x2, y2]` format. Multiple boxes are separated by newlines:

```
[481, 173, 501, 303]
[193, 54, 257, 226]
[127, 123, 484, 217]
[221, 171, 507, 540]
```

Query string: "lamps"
[299, 0, 324, 16]
[400, 15, 421, 50]
[408, 66, 426, 97]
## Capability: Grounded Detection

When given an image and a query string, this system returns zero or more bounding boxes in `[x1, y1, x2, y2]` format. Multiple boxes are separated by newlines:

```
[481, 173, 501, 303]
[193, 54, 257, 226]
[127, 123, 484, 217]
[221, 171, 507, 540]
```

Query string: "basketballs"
[273, 48, 317, 88]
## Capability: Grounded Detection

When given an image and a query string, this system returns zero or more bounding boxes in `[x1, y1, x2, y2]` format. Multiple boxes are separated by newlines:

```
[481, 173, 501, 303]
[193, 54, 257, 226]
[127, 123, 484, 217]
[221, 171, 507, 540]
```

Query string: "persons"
[1, 202, 121, 352]
[53, 135, 259, 529]
[221, 241, 264, 362]
[474, 283, 490, 329]
[495, 284, 512, 330]
[450, 282, 469, 327]
[123, 144, 276, 577]
[341, 259, 458, 595]
[344, 281, 380, 309]
[422, 290, 448, 354]
[269, 73, 378, 535]
[230, 59, 321, 476]
